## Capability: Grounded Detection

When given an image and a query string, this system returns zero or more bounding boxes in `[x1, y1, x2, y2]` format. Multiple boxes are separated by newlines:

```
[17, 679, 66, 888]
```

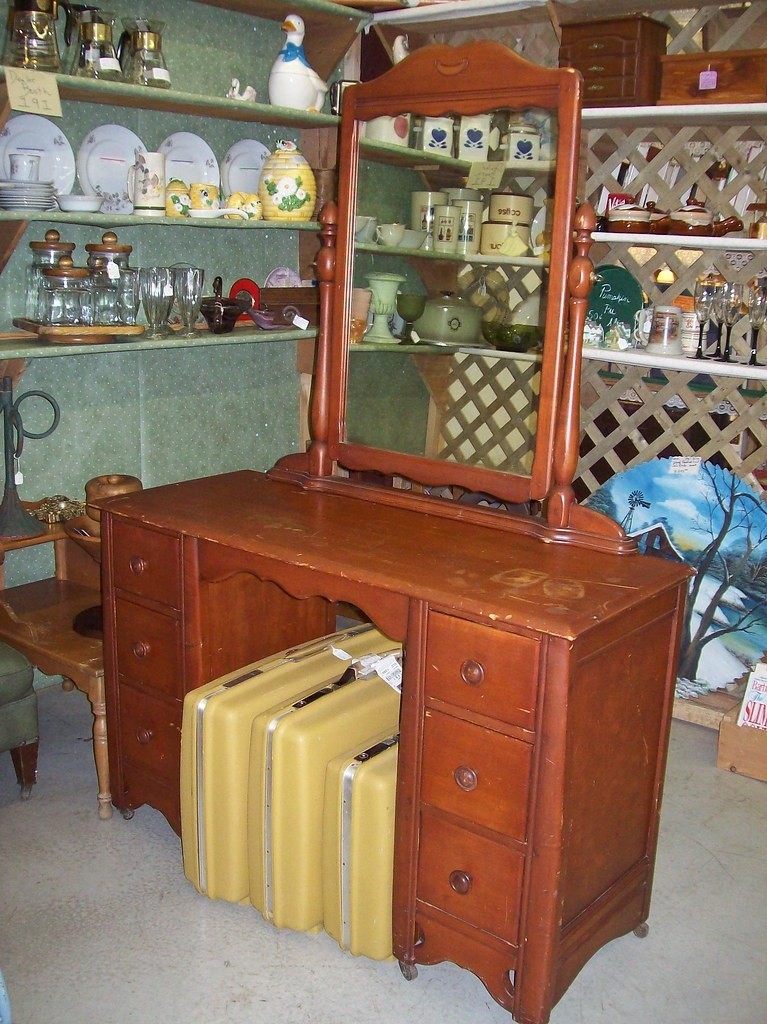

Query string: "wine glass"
[687, 280, 767, 367]
[394, 294, 426, 346]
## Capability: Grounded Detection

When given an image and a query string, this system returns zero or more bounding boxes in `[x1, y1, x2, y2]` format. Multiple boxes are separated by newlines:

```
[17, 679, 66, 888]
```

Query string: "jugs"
[0, 0, 170, 89]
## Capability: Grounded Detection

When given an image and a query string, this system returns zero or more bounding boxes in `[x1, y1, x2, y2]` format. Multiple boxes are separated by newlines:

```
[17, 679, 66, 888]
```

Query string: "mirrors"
[322, 37, 582, 505]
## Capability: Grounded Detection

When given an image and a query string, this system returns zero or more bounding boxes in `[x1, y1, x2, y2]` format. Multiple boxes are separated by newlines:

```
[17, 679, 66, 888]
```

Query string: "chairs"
[0, 472, 143, 819]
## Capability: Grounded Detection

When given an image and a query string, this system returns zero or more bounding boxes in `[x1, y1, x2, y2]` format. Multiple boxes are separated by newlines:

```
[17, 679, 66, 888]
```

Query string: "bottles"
[23, 228, 140, 326]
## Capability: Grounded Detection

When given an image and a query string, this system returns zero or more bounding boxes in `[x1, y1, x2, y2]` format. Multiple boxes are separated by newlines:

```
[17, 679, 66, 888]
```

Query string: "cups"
[139, 264, 204, 338]
[355, 186, 483, 256]
[635, 304, 711, 355]
[9, 153, 40, 181]
[349, 318, 367, 346]
[126, 151, 165, 215]
[351, 288, 371, 321]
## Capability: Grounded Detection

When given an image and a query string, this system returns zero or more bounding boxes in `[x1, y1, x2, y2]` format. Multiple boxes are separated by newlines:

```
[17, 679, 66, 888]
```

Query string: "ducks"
[269, 14, 329, 113]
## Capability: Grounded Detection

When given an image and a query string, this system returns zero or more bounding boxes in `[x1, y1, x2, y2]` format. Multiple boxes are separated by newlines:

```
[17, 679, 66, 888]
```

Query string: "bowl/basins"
[483, 321, 545, 354]
[54, 194, 107, 214]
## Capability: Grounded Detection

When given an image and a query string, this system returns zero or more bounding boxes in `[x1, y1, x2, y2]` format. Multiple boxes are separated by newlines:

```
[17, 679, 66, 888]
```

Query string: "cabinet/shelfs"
[355, 138, 555, 365]
[1, 0, 347, 598]
[561, 97, 766, 727]
[557, 12, 671, 107]
[84, 470, 699, 1024]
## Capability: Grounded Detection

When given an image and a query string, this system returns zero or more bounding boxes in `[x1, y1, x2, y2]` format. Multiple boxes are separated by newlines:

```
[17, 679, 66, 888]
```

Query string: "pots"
[413, 290, 484, 344]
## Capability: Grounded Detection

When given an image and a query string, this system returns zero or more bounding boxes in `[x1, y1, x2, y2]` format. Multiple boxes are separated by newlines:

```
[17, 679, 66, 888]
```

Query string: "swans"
[224, 78, 256, 102]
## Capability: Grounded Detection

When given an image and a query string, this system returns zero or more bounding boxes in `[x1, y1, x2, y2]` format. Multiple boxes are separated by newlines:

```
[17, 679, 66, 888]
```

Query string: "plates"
[1, 113, 149, 213]
[221, 139, 272, 196]
[229, 267, 301, 322]
[586, 265, 645, 336]
[157, 132, 220, 192]
